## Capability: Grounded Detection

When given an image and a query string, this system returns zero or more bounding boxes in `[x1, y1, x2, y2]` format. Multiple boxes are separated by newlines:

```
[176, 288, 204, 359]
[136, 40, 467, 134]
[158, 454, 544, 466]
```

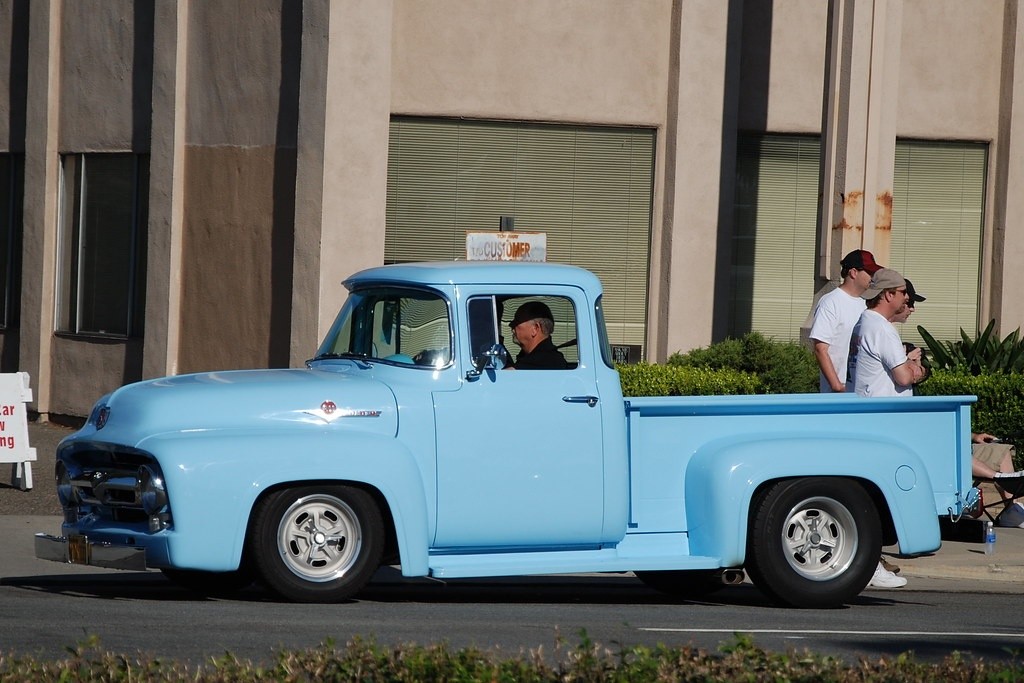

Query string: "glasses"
[889, 289, 907, 296]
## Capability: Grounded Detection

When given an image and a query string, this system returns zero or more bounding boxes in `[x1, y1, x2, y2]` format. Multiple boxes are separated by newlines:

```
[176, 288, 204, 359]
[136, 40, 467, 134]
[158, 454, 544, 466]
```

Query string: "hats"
[860, 269, 906, 299]
[842, 249, 883, 272]
[905, 278, 927, 302]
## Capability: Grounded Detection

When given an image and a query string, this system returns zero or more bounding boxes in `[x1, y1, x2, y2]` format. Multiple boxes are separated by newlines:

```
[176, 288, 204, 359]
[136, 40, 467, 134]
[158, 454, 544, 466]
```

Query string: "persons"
[808, 249, 1024, 587]
[412, 298, 569, 370]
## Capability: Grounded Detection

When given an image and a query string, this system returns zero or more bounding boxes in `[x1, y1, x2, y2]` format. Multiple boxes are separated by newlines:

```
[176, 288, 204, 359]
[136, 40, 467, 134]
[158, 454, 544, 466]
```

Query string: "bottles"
[985, 522, 997, 556]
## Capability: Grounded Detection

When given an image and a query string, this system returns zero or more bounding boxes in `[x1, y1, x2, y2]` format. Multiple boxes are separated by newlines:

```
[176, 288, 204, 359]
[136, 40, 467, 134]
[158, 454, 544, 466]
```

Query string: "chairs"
[972, 437, 1024, 526]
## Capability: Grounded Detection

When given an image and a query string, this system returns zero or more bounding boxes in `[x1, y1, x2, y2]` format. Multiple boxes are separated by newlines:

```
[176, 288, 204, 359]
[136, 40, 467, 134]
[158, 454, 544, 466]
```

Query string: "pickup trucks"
[33, 258, 982, 607]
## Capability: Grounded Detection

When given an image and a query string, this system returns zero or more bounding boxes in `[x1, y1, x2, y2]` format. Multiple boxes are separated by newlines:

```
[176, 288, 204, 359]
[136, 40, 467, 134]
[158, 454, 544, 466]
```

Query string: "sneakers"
[880, 554, 899, 573]
[867, 559, 907, 588]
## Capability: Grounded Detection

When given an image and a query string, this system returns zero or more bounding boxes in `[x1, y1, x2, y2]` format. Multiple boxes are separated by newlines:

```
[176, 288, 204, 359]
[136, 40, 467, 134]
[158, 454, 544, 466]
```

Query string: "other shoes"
[999, 502, 1024, 528]
[993, 470, 1024, 494]
